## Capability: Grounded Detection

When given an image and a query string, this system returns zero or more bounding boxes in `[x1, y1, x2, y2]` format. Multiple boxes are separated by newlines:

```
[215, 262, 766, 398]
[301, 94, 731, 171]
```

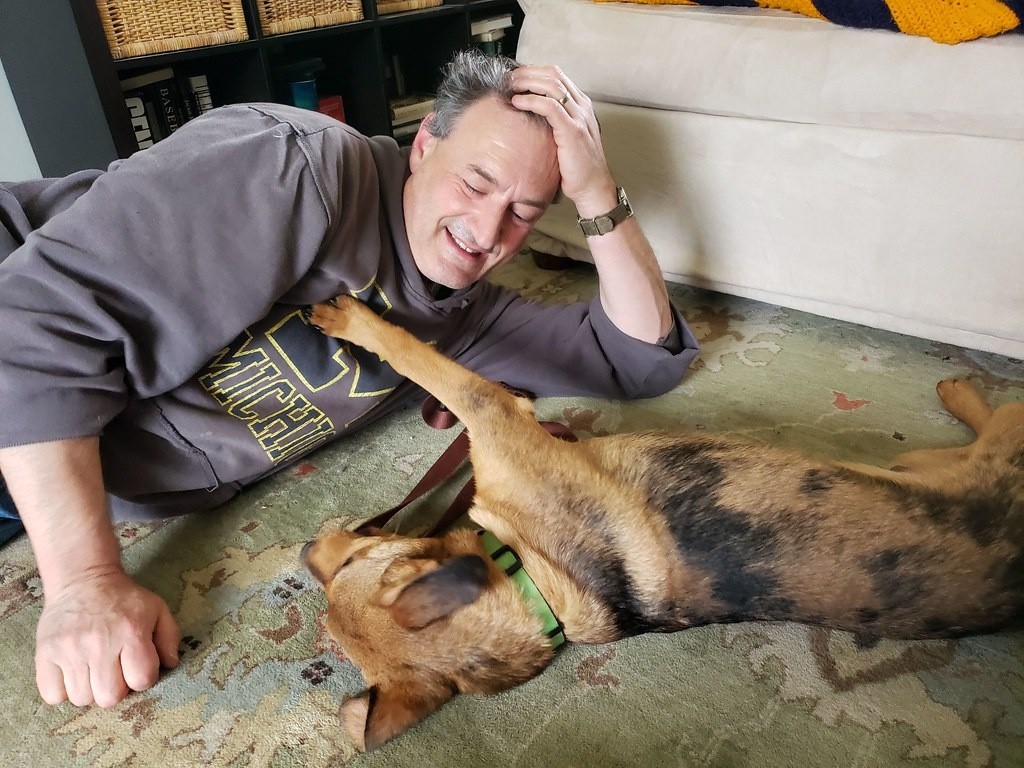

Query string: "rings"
[559, 91, 570, 105]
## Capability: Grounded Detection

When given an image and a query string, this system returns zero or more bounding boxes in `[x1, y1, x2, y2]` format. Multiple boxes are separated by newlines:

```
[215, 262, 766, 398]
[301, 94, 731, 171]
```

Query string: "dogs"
[298, 294, 1023, 754]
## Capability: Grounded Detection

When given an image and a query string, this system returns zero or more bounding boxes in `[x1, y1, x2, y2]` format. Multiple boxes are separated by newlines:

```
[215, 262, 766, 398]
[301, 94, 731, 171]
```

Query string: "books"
[118, 68, 214, 153]
[289, 79, 345, 125]
[391, 94, 437, 139]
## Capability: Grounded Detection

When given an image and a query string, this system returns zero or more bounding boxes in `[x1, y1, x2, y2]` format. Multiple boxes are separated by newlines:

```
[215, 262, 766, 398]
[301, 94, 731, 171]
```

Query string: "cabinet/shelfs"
[68, 0, 522, 159]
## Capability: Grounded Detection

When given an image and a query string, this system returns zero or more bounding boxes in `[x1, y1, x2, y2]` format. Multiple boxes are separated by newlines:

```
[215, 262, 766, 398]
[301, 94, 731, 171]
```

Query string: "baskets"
[96, 0, 249, 59]
[256, 0, 364, 37]
[376, 0, 444, 16]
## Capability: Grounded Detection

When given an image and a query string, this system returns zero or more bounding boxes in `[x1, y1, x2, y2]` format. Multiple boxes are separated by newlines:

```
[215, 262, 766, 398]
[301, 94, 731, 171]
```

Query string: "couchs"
[514, 0, 1024, 362]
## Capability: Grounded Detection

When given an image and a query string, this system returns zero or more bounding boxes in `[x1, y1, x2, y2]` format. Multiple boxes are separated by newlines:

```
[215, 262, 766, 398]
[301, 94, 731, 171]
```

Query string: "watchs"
[577, 186, 633, 237]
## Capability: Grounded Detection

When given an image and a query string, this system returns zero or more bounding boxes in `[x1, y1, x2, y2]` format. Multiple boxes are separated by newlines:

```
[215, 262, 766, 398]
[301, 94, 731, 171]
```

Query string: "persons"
[1, 52, 705, 710]
